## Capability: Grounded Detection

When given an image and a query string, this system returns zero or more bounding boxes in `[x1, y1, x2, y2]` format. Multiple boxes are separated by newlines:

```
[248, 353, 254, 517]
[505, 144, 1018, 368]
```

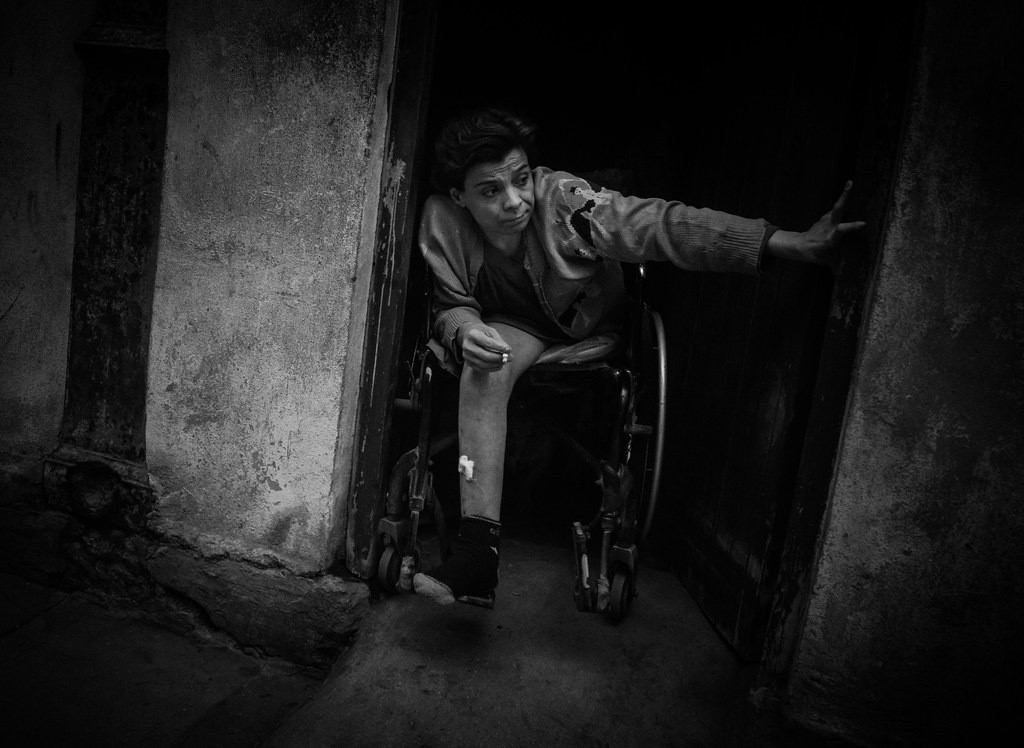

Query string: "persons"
[413, 106, 866, 608]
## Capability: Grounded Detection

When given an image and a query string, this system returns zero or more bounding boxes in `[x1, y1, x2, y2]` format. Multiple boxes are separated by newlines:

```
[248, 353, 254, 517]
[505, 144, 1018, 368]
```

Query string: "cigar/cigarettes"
[501, 352, 509, 362]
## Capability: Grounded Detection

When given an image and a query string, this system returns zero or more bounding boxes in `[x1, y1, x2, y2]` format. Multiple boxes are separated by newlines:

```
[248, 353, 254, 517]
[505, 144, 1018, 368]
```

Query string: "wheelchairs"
[360, 255, 671, 611]
